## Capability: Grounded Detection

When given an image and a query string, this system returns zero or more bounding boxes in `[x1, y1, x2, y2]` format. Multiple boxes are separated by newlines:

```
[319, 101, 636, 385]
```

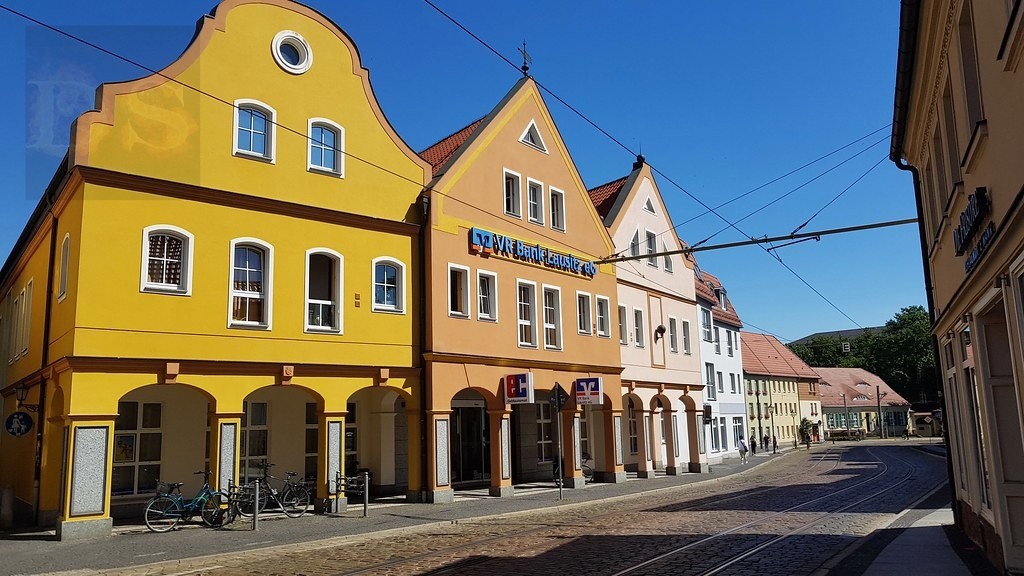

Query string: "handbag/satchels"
[744, 446, 748, 452]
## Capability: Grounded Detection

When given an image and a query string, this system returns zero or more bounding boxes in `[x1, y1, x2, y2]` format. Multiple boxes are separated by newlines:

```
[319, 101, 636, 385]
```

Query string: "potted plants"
[800, 418, 813, 445]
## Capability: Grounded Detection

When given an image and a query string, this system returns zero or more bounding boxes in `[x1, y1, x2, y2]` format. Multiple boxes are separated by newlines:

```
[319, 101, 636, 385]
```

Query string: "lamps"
[655, 324, 666, 343]
[15, 381, 38, 413]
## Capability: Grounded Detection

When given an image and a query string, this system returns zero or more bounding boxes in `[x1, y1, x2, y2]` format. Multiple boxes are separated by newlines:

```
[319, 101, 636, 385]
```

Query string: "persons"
[738, 435, 749, 464]
[764, 434, 770, 451]
[750, 435, 757, 456]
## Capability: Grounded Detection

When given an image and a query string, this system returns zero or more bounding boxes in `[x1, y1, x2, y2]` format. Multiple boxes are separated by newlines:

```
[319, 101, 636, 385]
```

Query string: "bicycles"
[554, 456, 593, 487]
[236, 458, 310, 518]
[144, 470, 235, 533]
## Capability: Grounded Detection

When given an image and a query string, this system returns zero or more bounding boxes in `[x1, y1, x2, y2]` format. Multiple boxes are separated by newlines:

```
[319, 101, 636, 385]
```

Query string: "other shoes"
[740, 463, 743, 464]
[745, 462, 748, 464]
